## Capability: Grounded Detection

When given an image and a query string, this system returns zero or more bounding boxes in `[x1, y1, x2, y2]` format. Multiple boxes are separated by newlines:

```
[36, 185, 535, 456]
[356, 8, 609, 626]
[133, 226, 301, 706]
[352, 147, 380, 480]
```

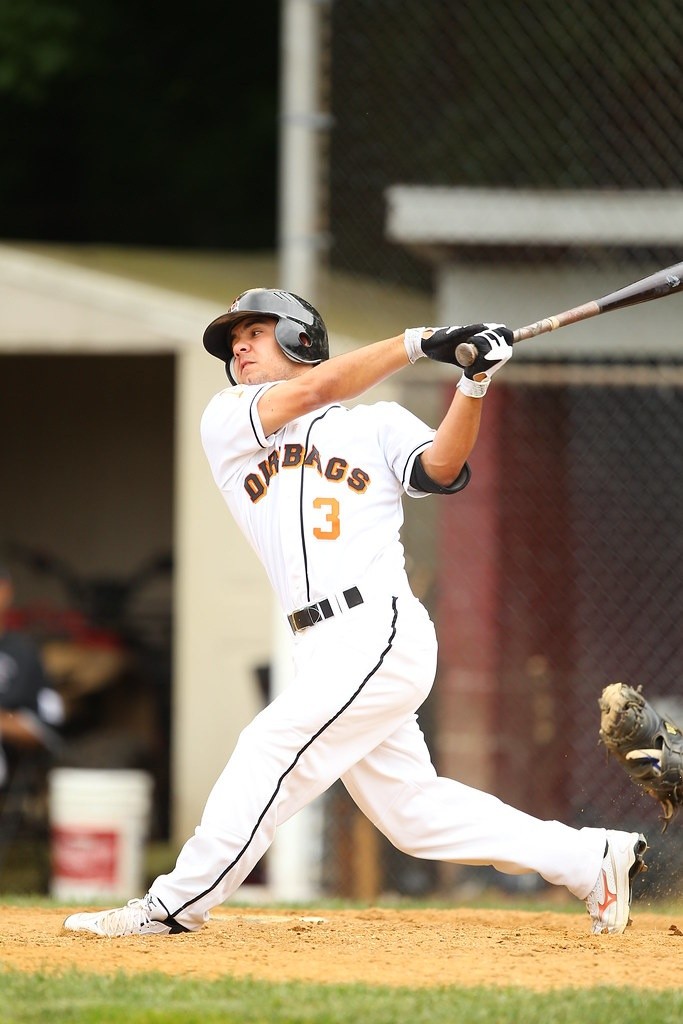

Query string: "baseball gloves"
[597, 681, 683, 840]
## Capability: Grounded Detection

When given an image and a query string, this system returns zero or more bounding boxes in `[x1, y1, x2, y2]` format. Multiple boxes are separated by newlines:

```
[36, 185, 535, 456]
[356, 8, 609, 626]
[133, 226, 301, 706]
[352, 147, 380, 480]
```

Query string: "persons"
[61, 288, 648, 938]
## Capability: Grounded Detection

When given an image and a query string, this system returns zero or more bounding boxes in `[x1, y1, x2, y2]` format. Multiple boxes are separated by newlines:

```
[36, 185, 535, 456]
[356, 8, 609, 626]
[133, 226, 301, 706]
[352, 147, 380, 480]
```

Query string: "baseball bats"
[453, 261, 683, 369]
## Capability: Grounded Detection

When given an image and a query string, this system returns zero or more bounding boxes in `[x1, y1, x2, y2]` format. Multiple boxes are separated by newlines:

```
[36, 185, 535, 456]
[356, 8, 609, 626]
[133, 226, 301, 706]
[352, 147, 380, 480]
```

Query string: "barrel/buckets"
[45, 766, 154, 908]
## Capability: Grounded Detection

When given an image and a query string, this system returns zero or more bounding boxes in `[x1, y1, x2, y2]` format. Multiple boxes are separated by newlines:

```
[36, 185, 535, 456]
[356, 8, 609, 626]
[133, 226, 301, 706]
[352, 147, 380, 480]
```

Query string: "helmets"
[203, 287, 329, 386]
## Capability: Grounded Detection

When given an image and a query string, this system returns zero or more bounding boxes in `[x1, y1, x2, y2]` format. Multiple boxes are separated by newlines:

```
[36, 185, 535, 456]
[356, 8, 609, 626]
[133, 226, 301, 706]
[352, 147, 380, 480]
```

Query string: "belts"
[287, 586, 364, 635]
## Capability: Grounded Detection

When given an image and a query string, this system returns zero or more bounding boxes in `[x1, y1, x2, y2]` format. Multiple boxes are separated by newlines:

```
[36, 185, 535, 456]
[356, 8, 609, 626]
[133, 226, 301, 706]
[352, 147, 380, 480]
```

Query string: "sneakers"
[582, 829, 647, 936]
[62, 892, 193, 940]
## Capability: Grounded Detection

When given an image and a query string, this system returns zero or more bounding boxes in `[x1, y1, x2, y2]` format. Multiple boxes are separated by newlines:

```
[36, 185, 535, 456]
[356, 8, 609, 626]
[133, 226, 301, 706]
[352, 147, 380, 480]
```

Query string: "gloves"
[403, 322, 506, 370]
[456, 327, 514, 399]
